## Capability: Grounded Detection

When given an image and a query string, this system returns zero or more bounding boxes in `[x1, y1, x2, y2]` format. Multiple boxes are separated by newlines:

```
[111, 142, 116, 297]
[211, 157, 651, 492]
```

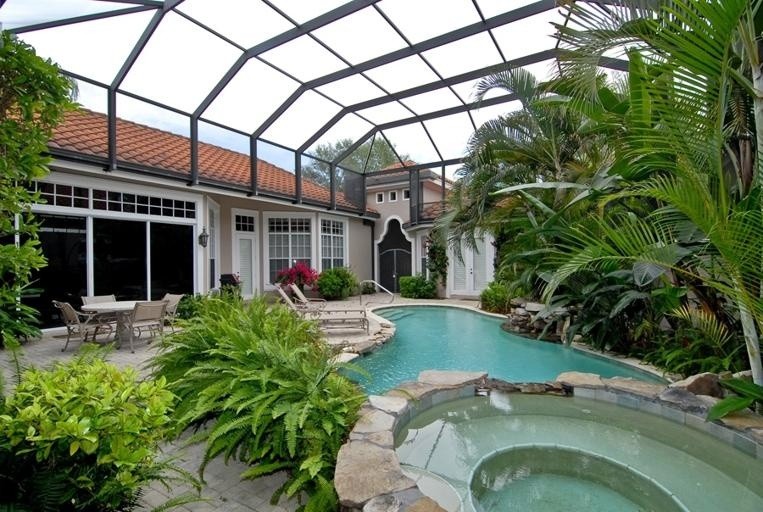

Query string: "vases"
[291, 291, 305, 300]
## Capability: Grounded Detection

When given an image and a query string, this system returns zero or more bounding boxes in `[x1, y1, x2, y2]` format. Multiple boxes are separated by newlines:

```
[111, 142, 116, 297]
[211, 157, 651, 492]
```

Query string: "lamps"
[198, 225, 211, 248]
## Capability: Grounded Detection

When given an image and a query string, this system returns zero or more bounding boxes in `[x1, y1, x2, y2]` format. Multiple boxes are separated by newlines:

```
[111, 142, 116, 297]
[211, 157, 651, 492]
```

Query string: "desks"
[80, 301, 153, 342]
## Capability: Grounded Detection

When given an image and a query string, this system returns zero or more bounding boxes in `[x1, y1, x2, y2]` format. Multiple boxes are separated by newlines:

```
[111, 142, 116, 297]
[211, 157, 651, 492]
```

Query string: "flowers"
[275, 261, 319, 289]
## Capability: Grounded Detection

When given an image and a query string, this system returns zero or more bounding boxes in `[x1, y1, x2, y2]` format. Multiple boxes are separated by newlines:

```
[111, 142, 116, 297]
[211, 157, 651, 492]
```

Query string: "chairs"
[152, 293, 184, 334]
[118, 300, 165, 353]
[53, 301, 101, 354]
[80, 294, 124, 339]
